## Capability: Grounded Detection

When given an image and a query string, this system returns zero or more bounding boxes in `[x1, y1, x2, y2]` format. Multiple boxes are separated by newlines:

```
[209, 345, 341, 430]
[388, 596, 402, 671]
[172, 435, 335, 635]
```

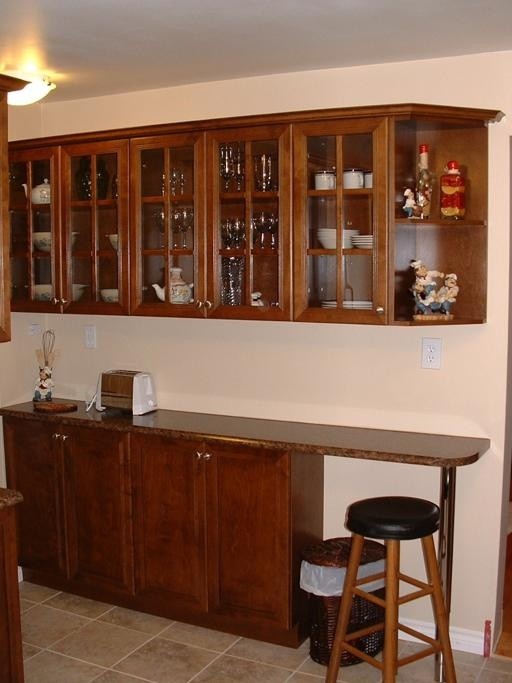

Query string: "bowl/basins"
[105, 233, 118, 250]
[32, 230, 81, 253]
[22, 283, 91, 301]
[101, 287, 150, 302]
[316, 227, 360, 248]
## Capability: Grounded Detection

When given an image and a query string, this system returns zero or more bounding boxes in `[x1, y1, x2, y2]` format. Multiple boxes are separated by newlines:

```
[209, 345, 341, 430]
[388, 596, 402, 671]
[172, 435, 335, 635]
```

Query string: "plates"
[320, 300, 374, 309]
[349, 234, 373, 249]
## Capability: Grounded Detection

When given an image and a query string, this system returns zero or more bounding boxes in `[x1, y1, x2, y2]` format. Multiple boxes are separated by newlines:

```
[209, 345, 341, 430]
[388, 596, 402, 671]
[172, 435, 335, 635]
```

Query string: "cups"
[150, 207, 165, 248]
[234, 148, 245, 192]
[220, 145, 235, 192]
[178, 207, 193, 249]
[255, 153, 272, 192]
[364, 169, 373, 188]
[159, 166, 187, 195]
[268, 210, 278, 249]
[314, 170, 337, 189]
[343, 168, 365, 188]
[221, 216, 258, 249]
[172, 207, 182, 248]
[256, 210, 272, 248]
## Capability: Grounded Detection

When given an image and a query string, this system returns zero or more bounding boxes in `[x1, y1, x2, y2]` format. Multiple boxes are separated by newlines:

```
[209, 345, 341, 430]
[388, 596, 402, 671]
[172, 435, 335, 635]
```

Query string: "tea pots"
[21, 178, 50, 203]
[152, 267, 195, 304]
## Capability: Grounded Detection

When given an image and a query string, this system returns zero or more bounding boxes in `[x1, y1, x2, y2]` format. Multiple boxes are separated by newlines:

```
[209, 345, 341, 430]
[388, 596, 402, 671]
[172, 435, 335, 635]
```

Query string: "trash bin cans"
[303, 537, 386, 667]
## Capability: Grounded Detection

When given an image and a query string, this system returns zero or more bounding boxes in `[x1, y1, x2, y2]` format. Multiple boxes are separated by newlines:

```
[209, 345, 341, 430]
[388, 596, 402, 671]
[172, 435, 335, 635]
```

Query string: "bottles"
[415, 146, 432, 217]
[221, 258, 240, 307]
[440, 161, 466, 220]
[238, 255, 244, 295]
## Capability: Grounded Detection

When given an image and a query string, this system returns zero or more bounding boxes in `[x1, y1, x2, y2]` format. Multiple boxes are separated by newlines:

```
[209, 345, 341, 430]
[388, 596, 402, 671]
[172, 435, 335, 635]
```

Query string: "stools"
[328, 497, 458, 682]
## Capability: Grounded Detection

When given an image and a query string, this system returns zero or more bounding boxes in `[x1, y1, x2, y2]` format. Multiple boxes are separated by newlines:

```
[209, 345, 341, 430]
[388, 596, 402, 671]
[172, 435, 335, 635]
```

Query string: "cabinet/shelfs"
[128, 125, 295, 324]
[292, 116, 416, 329]
[388, 112, 489, 326]
[3, 414, 134, 610]
[130, 432, 324, 649]
[9, 139, 127, 316]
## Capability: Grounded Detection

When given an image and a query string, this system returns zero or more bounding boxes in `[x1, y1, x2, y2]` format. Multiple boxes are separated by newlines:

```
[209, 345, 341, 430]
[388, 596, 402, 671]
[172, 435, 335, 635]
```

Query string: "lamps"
[6, 75, 59, 110]
